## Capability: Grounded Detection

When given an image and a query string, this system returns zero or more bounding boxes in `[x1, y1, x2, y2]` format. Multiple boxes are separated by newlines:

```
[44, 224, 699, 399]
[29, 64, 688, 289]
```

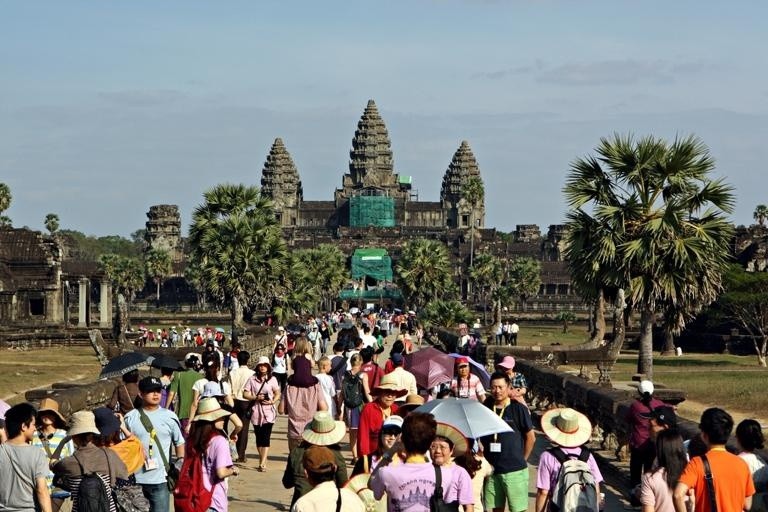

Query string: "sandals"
[259, 464, 267, 473]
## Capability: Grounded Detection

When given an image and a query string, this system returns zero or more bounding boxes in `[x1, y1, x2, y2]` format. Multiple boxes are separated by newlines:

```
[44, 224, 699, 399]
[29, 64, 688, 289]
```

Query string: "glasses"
[429, 445, 450, 451]
[698, 424, 703, 430]
[276, 348, 284, 350]
[379, 390, 400, 397]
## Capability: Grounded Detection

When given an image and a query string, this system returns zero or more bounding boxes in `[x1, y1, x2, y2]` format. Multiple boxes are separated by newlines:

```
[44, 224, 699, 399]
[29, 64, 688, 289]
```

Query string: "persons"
[0, 304, 768, 512]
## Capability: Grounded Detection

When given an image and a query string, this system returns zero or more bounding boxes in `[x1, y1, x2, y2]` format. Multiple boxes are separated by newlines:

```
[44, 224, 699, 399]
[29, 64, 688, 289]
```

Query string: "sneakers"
[237, 457, 247, 463]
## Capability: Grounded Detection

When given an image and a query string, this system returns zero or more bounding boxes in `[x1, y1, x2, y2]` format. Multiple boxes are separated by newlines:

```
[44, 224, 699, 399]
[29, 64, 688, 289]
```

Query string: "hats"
[302, 445, 336, 475]
[192, 397, 232, 422]
[368, 376, 409, 398]
[199, 380, 225, 401]
[455, 356, 470, 368]
[184, 352, 200, 365]
[36, 397, 67, 427]
[540, 406, 592, 448]
[430, 422, 469, 457]
[278, 326, 285, 333]
[66, 410, 102, 436]
[138, 375, 163, 395]
[381, 414, 404, 430]
[495, 355, 516, 369]
[388, 353, 404, 365]
[399, 394, 426, 412]
[637, 379, 654, 395]
[256, 356, 272, 368]
[638, 406, 678, 428]
[94, 407, 120, 437]
[300, 410, 347, 447]
[312, 324, 318, 329]
[341, 472, 388, 512]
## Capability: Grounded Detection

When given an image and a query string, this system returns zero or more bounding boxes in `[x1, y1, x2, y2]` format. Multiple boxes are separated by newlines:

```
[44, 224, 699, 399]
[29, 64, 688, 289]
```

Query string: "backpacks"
[76, 472, 110, 512]
[173, 453, 212, 511]
[549, 459, 600, 512]
[341, 376, 365, 409]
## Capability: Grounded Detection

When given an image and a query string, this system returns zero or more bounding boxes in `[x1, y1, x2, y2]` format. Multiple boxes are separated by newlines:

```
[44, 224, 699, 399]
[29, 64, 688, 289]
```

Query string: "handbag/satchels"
[245, 402, 254, 421]
[166, 466, 180, 492]
[112, 484, 151, 512]
[430, 495, 445, 512]
